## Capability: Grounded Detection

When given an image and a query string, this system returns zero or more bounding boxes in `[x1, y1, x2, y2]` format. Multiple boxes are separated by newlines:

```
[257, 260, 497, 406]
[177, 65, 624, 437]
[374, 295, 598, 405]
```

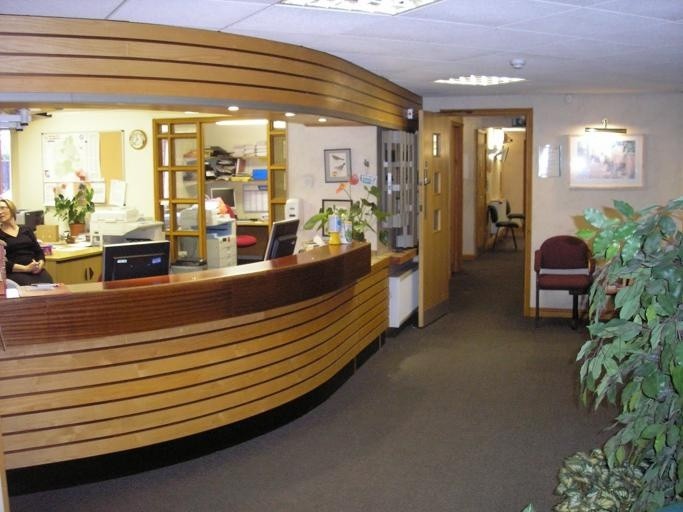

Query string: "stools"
[236, 234, 256, 248]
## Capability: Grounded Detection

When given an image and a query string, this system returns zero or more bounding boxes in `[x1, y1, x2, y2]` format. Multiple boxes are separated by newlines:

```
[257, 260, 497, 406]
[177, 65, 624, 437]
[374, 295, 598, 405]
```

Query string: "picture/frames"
[568, 134, 644, 189]
[324, 148, 352, 183]
[321, 200, 352, 237]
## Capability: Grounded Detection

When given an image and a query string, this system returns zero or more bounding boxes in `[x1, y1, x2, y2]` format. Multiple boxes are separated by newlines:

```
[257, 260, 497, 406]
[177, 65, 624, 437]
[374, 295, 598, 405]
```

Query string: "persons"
[1, 200, 53, 286]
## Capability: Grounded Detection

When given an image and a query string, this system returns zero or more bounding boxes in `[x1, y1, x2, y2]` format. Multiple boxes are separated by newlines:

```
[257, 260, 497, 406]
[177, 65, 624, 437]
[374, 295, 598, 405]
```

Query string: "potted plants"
[54, 182, 95, 236]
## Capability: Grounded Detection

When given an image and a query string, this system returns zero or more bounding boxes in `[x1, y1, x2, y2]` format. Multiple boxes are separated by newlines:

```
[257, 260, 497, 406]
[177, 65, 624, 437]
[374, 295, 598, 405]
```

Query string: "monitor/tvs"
[104, 240, 170, 281]
[264, 220, 300, 261]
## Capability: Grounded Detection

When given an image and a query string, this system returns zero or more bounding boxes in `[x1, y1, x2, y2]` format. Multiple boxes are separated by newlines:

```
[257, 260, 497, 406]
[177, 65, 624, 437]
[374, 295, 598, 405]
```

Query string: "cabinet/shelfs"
[183, 155, 268, 186]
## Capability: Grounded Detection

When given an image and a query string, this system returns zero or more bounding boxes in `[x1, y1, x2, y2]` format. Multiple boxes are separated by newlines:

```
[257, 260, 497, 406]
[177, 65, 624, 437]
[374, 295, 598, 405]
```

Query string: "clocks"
[128, 130, 146, 150]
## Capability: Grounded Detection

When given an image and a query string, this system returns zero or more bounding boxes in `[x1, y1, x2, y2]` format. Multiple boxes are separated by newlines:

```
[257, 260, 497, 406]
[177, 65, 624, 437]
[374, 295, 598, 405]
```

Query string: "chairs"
[487, 205, 518, 249]
[534, 236, 595, 325]
[504, 200, 524, 238]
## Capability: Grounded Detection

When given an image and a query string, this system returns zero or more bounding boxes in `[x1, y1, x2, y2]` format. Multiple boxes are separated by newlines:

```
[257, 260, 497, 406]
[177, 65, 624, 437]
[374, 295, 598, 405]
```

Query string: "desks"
[237, 218, 269, 227]
[36, 240, 103, 284]
